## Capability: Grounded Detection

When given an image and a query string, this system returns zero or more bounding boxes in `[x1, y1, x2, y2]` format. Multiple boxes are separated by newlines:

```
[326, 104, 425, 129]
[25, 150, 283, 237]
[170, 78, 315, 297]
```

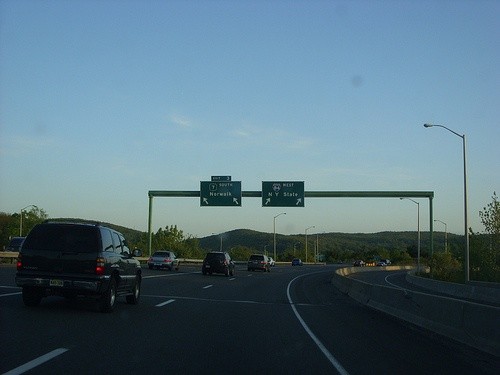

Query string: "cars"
[247, 253, 271, 273]
[353, 260, 366, 267]
[268, 257, 276, 267]
[291, 258, 303, 267]
[201, 249, 236, 277]
[14, 219, 144, 315]
[147, 250, 181, 272]
[380, 258, 392, 266]
[376, 262, 387, 267]
[6, 236, 27, 252]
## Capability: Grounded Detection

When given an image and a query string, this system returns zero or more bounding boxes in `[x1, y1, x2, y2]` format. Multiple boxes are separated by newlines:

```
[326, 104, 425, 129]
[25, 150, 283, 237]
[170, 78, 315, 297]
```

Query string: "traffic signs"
[261, 180, 305, 208]
[199, 180, 242, 207]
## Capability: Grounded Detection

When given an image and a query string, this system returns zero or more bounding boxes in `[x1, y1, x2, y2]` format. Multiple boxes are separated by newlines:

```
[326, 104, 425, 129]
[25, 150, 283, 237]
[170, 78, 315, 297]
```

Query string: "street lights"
[316, 232, 324, 262]
[423, 121, 470, 282]
[399, 197, 421, 273]
[264, 244, 271, 255]
[293, 242, 300, 258]
[273, 212, 287, 262]
[212, 232, 222, 251]
[433, 219, 448, 255]
[313, 238, 322, 262]
[18, 203, 37, 236]
[305, 225, 316, 263]
